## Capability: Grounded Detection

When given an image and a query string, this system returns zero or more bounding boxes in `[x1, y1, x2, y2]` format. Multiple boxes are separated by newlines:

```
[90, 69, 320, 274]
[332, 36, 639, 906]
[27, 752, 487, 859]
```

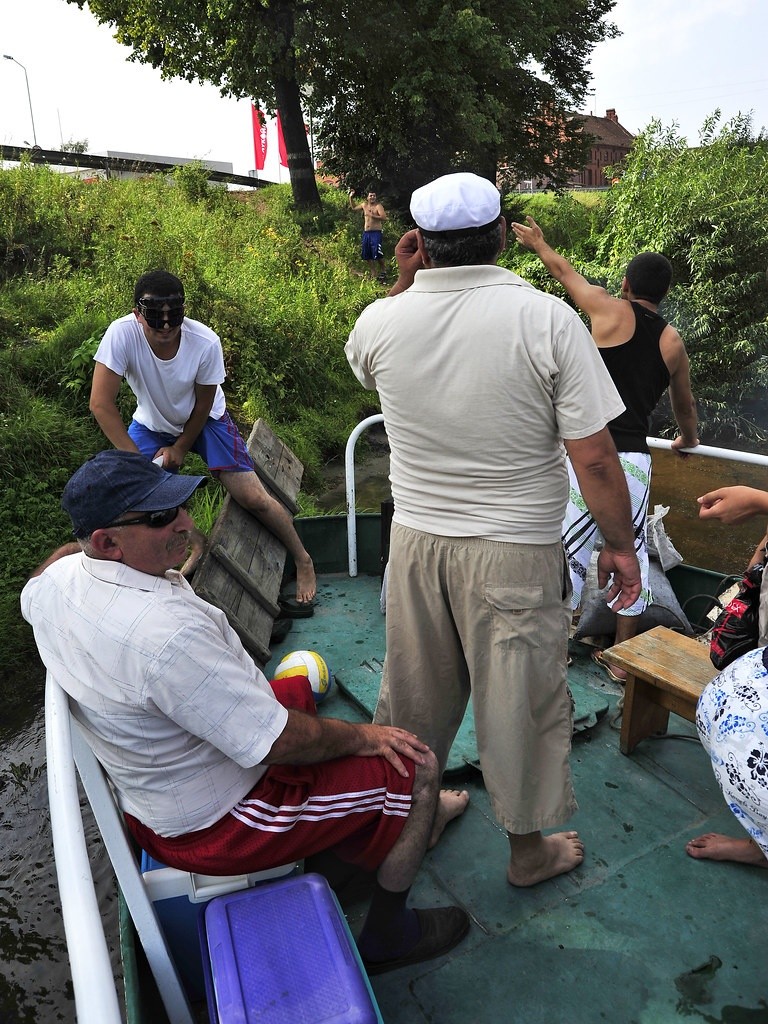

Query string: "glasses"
[138, 309, 186, 328]
[138, 294, 186, 309]
[137, 304, 185, 319]
[103, 497, 188, 529]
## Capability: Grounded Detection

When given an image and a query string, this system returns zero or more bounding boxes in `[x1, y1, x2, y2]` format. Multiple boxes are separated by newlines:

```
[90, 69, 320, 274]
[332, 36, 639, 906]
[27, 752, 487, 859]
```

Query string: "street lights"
[3, 54, 37, 149]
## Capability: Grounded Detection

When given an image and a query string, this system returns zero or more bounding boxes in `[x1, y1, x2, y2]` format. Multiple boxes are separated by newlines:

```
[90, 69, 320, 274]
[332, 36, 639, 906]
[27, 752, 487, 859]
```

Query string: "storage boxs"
[198, 873, 385, 1024]
[138, 848, 298, 1016]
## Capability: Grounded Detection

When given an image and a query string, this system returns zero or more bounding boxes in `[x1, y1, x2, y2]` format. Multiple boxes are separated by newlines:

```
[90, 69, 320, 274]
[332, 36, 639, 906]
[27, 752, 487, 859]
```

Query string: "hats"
[409, 173, 502, 239]
[61, 449, 210, 540]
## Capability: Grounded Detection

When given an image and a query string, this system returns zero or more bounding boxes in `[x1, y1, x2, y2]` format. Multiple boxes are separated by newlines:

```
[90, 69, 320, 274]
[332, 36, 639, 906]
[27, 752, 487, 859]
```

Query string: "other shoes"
[379, 271, 386, 279]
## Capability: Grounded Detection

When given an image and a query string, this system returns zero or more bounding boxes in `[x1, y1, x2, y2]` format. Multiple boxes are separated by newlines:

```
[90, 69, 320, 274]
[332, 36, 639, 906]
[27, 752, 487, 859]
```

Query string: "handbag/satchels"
[710, 563, 765, 671]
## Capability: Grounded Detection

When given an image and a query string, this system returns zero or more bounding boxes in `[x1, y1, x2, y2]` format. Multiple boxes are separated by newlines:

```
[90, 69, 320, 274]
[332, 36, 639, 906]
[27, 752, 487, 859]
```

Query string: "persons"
[335, 166, 644, 889]
[692, 644, 768, 863]
[347, 187, 387, 280]
[83, 271, 318, 605]
[508, 213, 704, 688]
[685, 478, 768, 871]
[18, 448, 472, 986]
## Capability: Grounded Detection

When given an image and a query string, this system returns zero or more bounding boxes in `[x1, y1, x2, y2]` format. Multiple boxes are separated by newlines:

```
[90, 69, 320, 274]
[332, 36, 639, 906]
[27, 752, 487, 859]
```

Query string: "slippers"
[362, 906, 470, 976]
[268, 618, 293, 644]
[277, 600, 314, 619]
[590, 649, 627, 684]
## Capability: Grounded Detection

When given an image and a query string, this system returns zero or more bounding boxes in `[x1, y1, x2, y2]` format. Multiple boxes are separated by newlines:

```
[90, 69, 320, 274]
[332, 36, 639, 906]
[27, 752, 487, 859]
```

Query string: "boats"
[42, 409, 768, 1024]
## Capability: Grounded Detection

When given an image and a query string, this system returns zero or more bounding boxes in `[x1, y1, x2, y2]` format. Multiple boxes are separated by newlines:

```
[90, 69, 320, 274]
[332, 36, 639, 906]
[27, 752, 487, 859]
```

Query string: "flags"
[276, 108, 288, 169]
[250, 103, 267, 171]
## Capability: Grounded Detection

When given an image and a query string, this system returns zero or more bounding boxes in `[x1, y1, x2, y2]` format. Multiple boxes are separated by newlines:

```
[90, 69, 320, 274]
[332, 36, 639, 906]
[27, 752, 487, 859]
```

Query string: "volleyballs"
[273, 651, 330, 703]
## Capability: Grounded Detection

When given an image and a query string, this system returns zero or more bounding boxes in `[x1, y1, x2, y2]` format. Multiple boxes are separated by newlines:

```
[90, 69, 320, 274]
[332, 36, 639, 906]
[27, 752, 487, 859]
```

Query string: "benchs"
[600, 625, 724, 756]
